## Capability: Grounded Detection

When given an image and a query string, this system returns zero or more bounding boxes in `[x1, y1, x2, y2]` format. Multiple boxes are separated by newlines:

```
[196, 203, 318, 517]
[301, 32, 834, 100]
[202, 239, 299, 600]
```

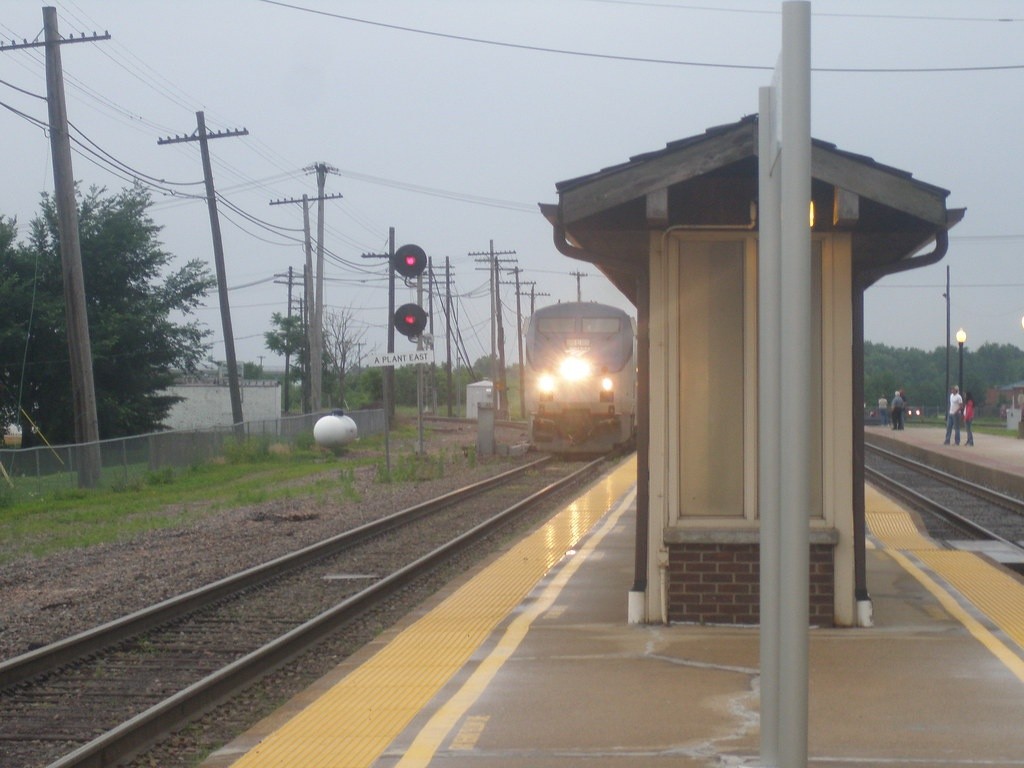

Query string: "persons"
[879, 395, 888, 425]
[893, 387, 907, 430]
[943, 385, 963, 445]
[962, 391, 975, 446]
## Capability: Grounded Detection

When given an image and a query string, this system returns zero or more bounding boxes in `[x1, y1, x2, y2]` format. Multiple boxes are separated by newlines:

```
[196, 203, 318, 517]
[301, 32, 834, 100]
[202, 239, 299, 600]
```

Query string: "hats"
[951, 384, 959, 392]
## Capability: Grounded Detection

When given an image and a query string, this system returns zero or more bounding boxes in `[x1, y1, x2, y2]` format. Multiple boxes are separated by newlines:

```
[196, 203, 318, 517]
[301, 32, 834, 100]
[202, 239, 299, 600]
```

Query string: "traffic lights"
[395, 244, 426, 278]
[395, 304, 428, 338]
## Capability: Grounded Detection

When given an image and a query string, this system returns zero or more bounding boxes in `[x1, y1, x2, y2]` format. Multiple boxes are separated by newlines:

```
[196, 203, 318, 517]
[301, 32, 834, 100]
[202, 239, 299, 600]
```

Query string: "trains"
[519, 300, 638, 452]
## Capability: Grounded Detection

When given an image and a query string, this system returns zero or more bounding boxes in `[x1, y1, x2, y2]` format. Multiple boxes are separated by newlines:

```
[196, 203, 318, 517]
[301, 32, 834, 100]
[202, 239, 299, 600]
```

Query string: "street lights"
[955, 328, 967, 415]
